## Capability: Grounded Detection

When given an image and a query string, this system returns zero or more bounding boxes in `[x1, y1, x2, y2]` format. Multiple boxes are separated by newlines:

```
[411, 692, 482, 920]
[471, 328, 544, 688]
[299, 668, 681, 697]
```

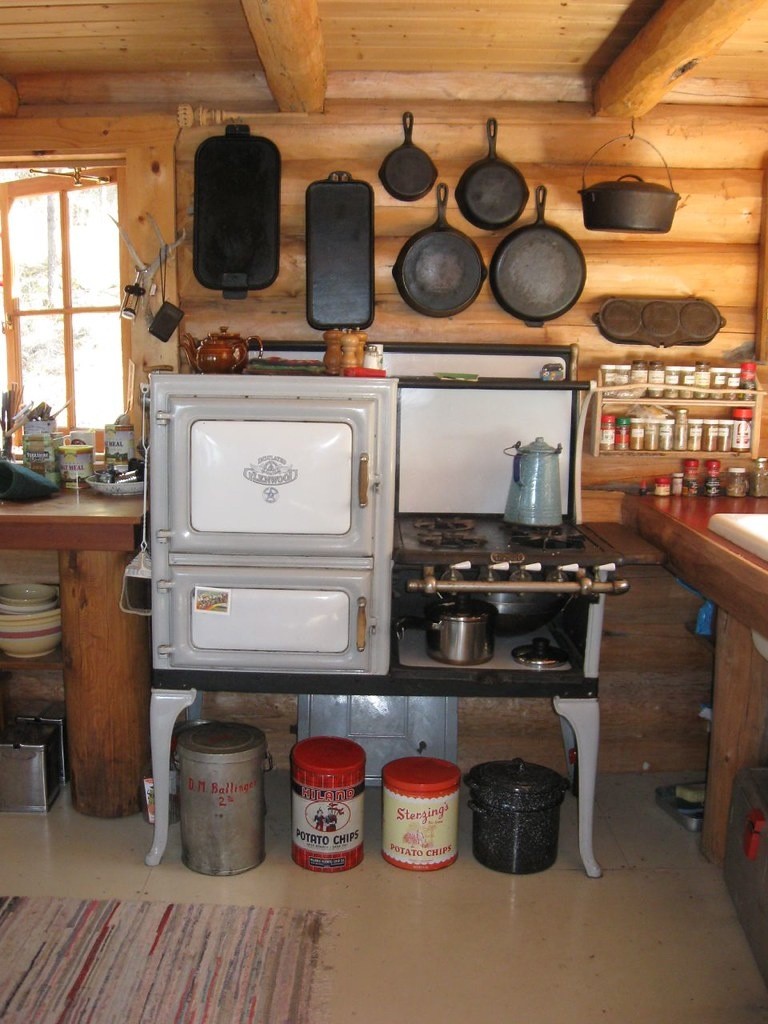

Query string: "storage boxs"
[17, 697, 67, 784]
[719, 762, 768, 988]
[0, 724, 60, 813]
[21, 430, 65, 486]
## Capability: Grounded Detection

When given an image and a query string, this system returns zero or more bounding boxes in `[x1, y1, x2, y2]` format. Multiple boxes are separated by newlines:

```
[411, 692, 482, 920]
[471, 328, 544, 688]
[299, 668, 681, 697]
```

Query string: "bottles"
[600, 407, 751, 451]
[362, 341, 385, 369]
[640, 456, 768, 498]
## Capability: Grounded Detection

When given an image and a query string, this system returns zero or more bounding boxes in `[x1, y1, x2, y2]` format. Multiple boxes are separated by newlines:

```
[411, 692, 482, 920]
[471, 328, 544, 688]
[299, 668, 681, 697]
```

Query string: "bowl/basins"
[0, 584, 62, 658]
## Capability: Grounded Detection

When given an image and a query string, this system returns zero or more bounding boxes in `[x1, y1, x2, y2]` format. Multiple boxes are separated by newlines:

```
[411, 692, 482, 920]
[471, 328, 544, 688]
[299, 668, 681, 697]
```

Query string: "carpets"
[0, 893, 331, 1024]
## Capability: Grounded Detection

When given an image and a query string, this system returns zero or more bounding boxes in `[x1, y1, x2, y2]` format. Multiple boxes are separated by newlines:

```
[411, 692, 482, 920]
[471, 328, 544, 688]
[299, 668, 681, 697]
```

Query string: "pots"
[194, 111, 727, 349]
[463, 758, 570, 873]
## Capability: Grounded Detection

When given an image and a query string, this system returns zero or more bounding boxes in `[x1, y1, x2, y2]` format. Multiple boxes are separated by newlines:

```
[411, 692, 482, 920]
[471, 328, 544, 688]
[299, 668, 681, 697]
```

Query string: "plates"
[85, 465, 144, 495]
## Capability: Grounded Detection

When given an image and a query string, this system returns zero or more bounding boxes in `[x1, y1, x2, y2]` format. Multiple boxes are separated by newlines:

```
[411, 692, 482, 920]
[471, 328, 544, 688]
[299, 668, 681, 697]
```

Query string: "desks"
[142, 673, 620, 880]
[637, 491, 768, 867]
[0, 482, 150, 820]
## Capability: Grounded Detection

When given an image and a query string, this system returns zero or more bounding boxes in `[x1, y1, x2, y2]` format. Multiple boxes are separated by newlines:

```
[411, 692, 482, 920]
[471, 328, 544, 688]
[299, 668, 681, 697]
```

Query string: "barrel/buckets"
[382, 757, 462, 872]
[288, 736, 367, 874]
[173, 721, 274, 878]
[22, 431, 94, 490]
[24, 420, 56, 434]
[104, 425, 135, 465]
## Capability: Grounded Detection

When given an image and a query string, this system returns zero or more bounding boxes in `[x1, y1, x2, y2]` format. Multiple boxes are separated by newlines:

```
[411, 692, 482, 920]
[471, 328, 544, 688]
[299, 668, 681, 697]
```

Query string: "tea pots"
[177, 326, 263, 374]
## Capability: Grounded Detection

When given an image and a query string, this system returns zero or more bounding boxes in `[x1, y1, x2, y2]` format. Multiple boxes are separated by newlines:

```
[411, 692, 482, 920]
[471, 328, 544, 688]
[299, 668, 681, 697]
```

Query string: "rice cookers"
[425, 596, 499, 665]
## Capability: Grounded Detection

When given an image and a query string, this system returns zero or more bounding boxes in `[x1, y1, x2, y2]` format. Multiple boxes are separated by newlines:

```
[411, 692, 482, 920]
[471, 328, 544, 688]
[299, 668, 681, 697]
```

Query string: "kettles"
[503, 437, 562, 528]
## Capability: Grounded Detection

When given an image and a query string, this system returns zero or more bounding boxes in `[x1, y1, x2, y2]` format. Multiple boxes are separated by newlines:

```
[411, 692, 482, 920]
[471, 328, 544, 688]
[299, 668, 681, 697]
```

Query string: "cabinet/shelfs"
[589, 358, 763, 462]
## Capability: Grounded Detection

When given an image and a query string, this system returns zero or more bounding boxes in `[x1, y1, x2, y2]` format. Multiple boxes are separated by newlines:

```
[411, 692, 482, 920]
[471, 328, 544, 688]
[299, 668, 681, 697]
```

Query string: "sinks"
[707, 512, 768, 562]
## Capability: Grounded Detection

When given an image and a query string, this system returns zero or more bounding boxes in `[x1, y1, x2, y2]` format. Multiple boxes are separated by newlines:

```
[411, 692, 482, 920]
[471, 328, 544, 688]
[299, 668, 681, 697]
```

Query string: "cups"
[391, 512, 630, 595]
[62, 431, 96, 463]
[601, 360, 757, 399]
